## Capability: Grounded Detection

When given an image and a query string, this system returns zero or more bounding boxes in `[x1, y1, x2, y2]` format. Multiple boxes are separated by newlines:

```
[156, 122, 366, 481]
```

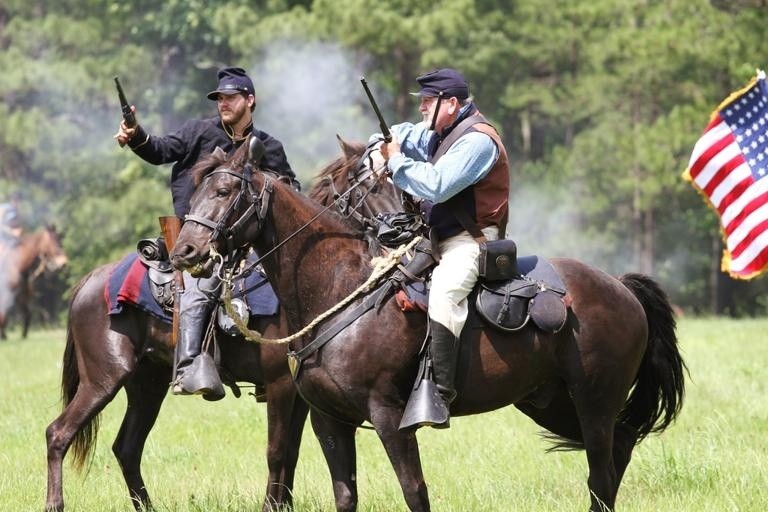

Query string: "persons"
[116, 66, 300, 395]
[0, 189, 24, 254]
[368, 67, 509, 429]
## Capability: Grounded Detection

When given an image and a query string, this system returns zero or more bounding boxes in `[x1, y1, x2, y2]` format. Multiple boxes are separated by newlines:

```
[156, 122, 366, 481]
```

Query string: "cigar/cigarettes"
[112, 132, 121, 142]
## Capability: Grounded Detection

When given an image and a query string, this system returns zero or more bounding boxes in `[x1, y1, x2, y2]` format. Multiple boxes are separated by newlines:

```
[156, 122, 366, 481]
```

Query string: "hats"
[206, 67, 256, 100]
[409, 67, 469, 100]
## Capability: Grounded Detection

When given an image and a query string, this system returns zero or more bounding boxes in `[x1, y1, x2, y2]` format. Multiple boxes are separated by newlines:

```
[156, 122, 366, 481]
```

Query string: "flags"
[680, 70, 767, 282]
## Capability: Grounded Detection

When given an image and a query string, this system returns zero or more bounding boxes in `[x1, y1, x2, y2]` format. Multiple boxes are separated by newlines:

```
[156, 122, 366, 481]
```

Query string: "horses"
[169, 131, 695, 512]
[0, 220, 70, 340]
[45, 133, 420, 512]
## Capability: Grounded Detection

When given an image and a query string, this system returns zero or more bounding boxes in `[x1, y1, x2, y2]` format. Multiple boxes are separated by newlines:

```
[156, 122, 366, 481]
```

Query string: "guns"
[114, 77, 136, 148]
[361, 78, 414, 211]
[159, 215, 182, 387]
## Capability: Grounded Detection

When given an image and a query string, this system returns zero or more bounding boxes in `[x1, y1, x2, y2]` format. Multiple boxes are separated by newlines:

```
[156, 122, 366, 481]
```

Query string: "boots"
[171, 301, 210, 396]
[422, 321, 460, 430]
